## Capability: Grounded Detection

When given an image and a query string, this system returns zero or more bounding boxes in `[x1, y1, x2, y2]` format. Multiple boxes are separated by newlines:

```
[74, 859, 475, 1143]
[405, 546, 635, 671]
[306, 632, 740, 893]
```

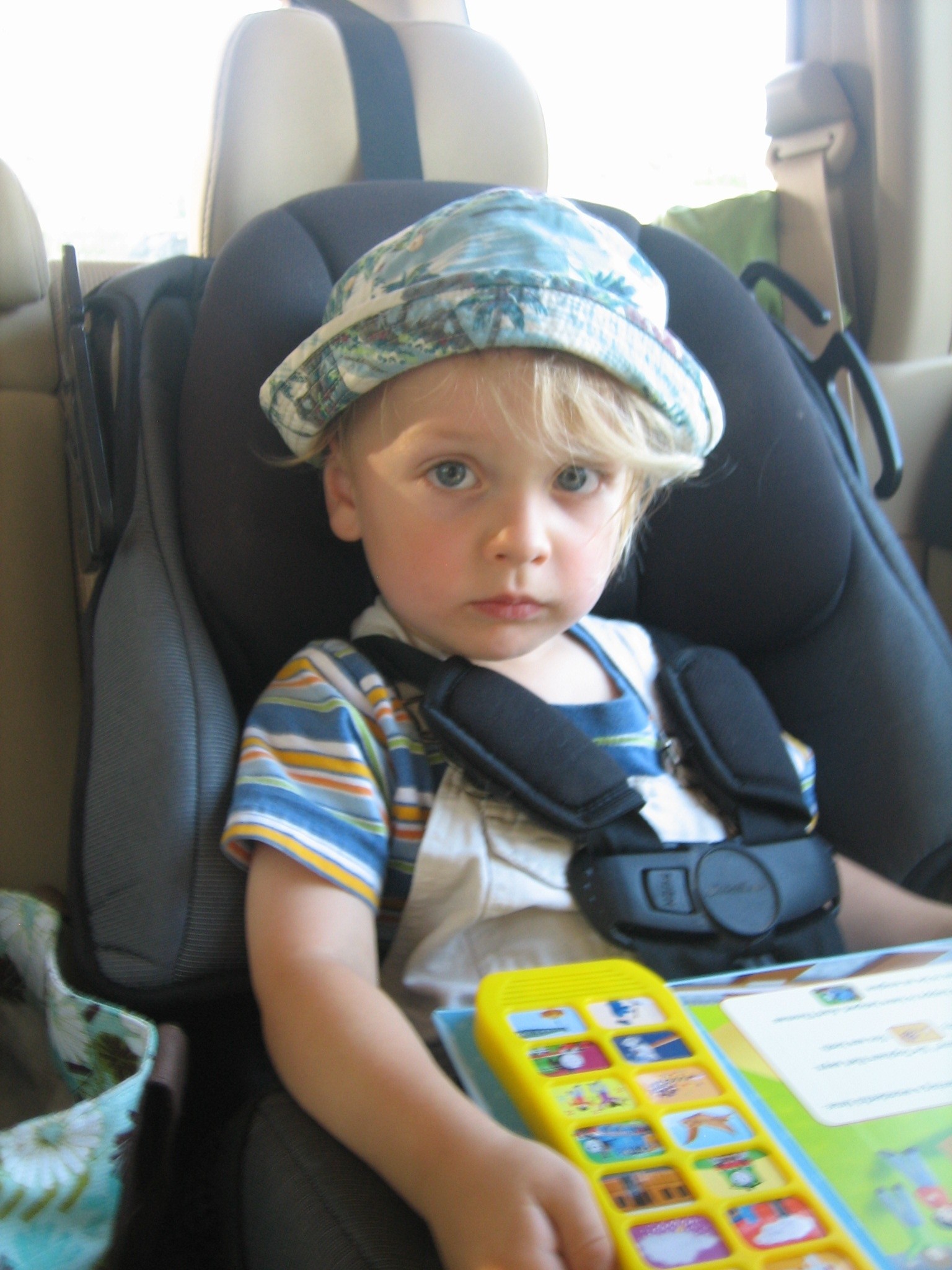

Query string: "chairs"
[50, 175, 952, 1270]
[0, 1, 551, 1270]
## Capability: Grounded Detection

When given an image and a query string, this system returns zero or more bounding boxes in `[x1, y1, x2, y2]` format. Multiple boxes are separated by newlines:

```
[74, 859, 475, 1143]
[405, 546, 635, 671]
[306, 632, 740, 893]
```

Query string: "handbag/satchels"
[1, 889, 158, 1269]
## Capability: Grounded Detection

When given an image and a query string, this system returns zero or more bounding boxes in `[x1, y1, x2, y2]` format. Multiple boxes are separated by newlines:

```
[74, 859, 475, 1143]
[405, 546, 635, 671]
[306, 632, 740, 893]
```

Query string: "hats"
[258, 186, 724, 462]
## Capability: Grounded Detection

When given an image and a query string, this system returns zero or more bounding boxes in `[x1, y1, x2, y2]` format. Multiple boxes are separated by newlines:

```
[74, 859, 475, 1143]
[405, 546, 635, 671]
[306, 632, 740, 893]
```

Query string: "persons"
[215, 184, 952, 1270]
